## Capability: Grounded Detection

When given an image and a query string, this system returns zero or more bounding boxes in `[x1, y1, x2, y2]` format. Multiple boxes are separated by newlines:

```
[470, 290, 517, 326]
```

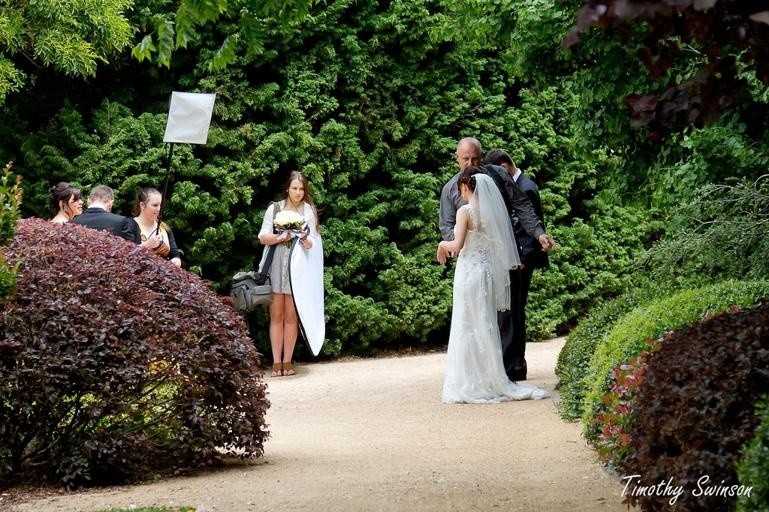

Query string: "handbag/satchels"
[229, 269, 274, 312]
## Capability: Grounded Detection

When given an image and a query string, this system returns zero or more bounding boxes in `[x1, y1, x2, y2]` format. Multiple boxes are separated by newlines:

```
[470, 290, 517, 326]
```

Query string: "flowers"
[272, 210, 306, 249]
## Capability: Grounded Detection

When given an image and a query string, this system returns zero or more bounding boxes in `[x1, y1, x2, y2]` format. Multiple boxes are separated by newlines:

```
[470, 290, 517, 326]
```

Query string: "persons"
[482, 148, 551, 381]
[437, 165, 499, 405]
[258, 170, 319, 377]
[47, 181, 85, 226]
[64, 183, 137, 245]
[127, 187, 182, 270]
[439, 137, 560, 389]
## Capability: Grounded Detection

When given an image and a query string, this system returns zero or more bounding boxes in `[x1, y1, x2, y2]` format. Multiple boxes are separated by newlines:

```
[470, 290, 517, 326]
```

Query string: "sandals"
[269, 361, 295, 377]
